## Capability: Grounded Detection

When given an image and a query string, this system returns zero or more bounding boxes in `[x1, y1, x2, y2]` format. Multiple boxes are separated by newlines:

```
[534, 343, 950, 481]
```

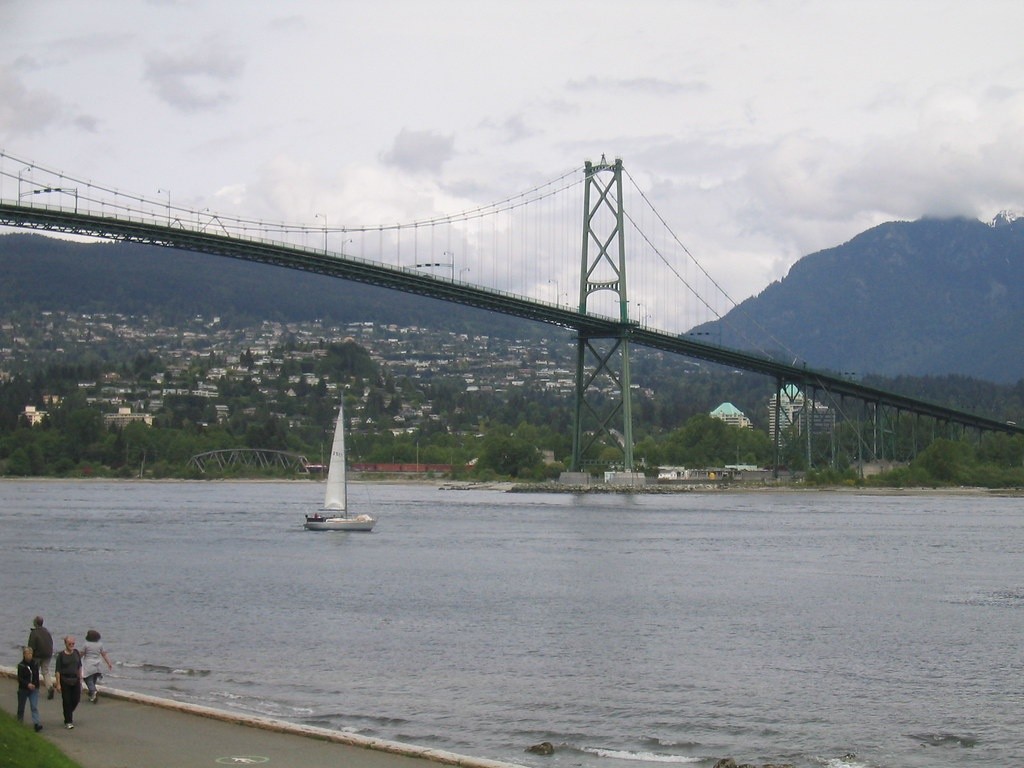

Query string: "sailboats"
[303, 391, 382, 530]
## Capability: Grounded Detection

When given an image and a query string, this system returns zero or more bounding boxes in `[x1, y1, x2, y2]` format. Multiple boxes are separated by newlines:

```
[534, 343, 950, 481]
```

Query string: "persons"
[55, 636, 83, 730]
[16, 645, 43, 732]
[80, 630, 112, 703]
[28, 616, 55, 700]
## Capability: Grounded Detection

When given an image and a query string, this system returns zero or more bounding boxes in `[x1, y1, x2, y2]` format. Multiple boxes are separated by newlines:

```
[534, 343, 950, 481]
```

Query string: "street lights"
[18, 166, 33, 207]
[341, 237, 354, 258]
[157, 187, 171, 228]
[459, 266, 471, 286]
[197, 207, 209, 232]
[442, 251, 454, 284]
[315, 213, 328, 256]
[549, 279, 559, 310]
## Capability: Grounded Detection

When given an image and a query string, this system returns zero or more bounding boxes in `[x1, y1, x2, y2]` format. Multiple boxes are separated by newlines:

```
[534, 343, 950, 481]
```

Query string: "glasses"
[66, 642, 75, 646]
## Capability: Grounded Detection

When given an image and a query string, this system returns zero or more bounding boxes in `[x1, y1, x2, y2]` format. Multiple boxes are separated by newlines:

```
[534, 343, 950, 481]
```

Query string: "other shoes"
[90, 691, 99, 703]
[65, 722, 74, 728]
[48, 688, 54, 699]
[34, 724, 43, 732]
